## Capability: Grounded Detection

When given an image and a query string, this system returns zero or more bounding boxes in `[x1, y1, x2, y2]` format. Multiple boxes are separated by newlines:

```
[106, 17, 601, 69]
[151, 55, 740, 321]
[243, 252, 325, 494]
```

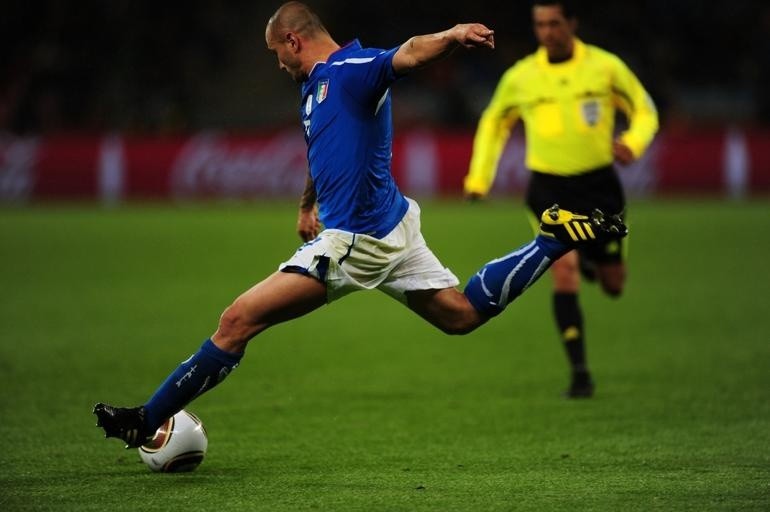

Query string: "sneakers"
[568, 370, 594, 399]
[93, 403, 156, 448]
[538, 204, 628, 251]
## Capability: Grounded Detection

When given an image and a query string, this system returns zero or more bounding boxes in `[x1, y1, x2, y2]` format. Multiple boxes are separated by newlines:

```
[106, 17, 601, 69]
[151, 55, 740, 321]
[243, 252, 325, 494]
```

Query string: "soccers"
[138, 408, 207, 473]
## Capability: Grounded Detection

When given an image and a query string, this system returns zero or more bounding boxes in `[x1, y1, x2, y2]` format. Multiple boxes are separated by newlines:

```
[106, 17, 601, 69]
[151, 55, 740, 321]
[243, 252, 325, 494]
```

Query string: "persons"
[464, 0, 660, 398]
[94, 0, 627, 452]
